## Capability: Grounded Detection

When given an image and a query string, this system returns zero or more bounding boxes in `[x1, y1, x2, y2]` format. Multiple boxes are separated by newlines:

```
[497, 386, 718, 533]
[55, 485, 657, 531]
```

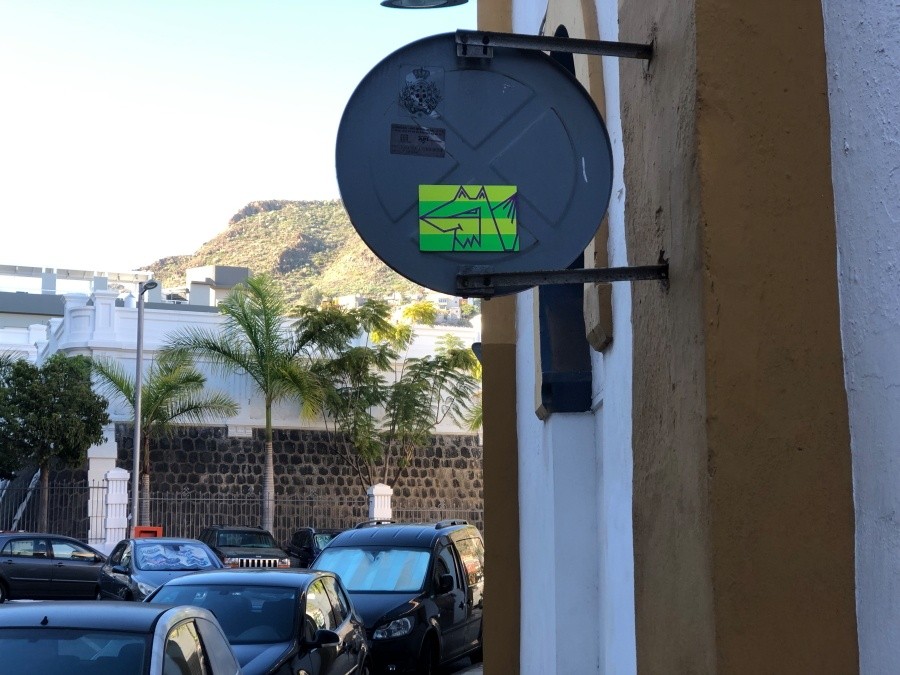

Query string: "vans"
[309, 520, 486, 674]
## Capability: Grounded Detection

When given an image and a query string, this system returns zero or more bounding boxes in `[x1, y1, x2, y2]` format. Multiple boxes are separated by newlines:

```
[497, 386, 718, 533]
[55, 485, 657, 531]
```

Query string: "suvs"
[287, 527, 351, 568]
[197, 524, 291, 569]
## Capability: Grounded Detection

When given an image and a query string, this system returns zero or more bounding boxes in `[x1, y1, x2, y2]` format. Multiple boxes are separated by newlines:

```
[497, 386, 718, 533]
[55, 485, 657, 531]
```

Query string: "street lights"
[132, 279, 157, 535]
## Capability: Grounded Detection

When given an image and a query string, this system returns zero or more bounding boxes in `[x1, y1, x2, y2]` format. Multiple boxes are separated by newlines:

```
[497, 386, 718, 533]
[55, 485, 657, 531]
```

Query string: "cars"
[142, 569, 367, 674]
[96, 538, 226, 602]
[0, 531, 110, 603]
[0, 598, 241, 674]
